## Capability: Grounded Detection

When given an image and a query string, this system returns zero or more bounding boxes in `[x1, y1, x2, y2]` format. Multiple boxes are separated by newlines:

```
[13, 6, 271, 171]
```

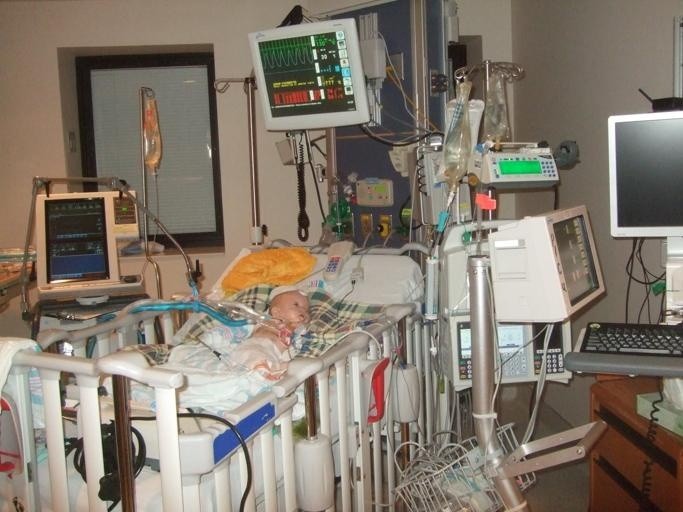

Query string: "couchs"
[1, 243, 437, 512]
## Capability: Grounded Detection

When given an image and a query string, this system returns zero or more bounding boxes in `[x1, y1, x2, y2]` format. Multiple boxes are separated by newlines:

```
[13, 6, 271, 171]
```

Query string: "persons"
[247, 290, 311, 379]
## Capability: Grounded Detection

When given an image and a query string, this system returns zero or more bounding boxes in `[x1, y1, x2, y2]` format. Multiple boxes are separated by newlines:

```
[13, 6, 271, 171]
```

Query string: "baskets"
[390, 422, 537, 512]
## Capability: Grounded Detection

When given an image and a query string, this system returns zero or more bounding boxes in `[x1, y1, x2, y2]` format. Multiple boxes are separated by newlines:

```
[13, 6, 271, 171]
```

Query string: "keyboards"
[563, 321, 683, 378]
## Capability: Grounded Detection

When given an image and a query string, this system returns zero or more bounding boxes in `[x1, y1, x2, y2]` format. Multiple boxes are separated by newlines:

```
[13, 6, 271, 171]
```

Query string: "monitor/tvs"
[34, 190, 147, 302]
[608, 110, 683, 238]
[488, 203, 606, 325]
[247, 17, 372, 133]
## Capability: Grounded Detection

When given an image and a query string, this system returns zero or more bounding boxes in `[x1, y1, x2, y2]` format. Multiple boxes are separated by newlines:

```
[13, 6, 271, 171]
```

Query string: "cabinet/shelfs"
[588, 377, 683, 510]
